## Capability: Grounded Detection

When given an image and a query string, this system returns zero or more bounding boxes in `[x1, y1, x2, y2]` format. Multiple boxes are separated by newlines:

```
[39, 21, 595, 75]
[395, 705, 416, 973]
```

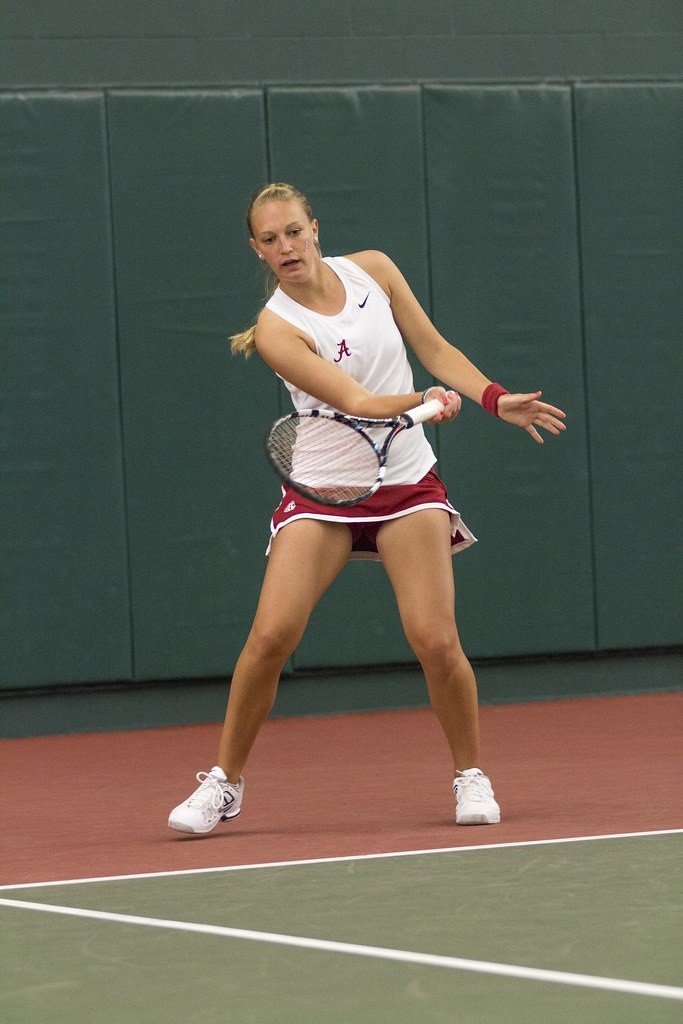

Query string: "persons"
[168, 184, 567, 834]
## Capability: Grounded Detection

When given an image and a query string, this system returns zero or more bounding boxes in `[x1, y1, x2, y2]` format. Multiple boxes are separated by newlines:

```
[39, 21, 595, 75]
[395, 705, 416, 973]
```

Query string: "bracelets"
[421, 386, 434, 404]
[481, 383, 510, 417]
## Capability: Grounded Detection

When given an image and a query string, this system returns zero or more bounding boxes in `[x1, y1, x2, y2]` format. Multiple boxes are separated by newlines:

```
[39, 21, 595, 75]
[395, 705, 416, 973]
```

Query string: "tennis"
[262, 397, 445, 509]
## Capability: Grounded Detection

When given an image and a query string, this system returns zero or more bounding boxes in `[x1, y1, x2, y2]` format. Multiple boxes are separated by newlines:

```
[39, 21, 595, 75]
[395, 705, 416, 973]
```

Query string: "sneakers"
[167, 765, 245, 833]
[452, 768, 501, 825]
